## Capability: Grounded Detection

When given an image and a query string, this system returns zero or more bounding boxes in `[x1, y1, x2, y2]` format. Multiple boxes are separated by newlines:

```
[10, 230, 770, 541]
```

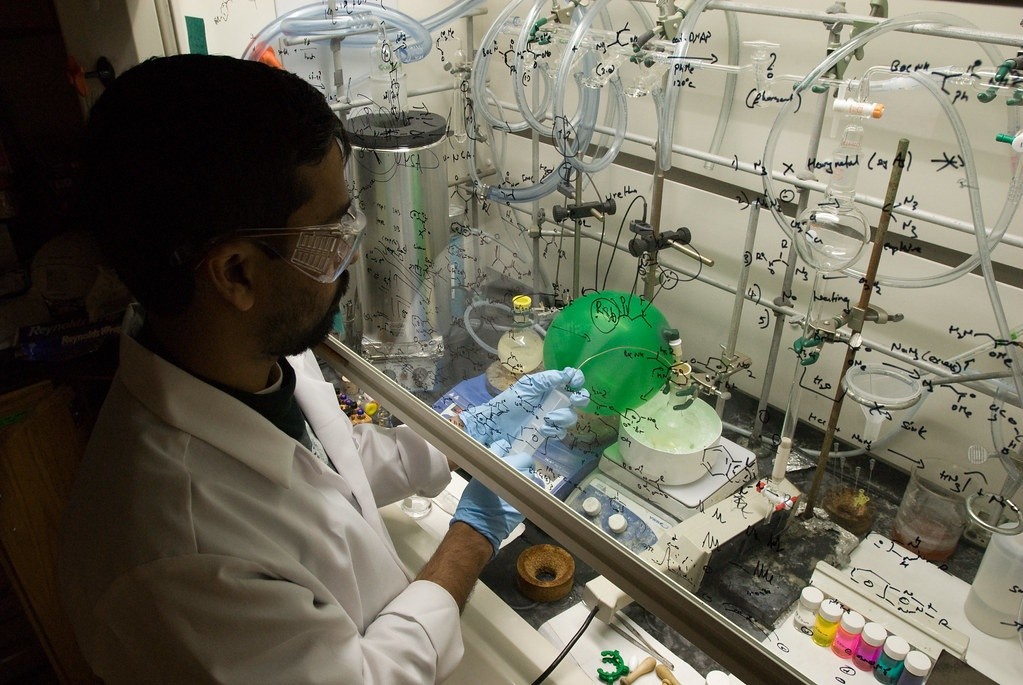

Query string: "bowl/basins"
[618, 391, 722, 485]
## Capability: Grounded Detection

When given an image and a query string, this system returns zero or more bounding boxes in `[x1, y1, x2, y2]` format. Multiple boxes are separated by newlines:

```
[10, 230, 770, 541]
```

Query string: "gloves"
[449, 438, 545, 563]
[458, 367, 591, 447]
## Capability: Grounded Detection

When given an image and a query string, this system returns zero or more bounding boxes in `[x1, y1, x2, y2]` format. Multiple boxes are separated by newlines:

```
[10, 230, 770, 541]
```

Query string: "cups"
[892, 458, 981, 562]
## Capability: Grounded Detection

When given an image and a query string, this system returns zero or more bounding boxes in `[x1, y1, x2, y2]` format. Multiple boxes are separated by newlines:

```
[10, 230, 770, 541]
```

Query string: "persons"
[60, 55, 590, 685]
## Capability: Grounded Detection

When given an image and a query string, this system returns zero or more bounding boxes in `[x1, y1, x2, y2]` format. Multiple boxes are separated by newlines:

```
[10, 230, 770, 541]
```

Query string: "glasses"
[192, 195, 368, 284]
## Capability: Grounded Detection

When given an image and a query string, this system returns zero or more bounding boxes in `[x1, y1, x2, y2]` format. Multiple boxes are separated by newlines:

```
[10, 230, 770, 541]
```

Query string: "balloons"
[543, 289, 676, 417]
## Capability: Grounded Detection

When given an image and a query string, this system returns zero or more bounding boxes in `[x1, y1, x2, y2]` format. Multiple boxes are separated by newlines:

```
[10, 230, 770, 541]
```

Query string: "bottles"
[812, 599, 844, 647]
[964, 522, 1023, 638]
[897, 651, 931, 685]
[793, 587, 824, 634]
[648, 362, 700, 449]
[852, 622, 887, 671]
[873, 636, 910, 685]
[498, 295, 543, 374]
[831, 611, 865, 659]
